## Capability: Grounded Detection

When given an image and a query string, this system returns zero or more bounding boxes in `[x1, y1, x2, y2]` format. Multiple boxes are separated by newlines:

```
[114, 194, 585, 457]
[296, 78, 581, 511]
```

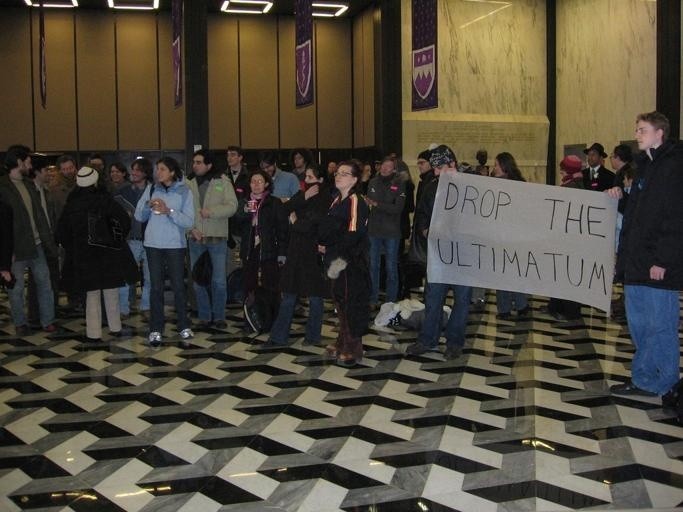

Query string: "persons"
[395, 161, 414, 300]
[0, 145, 60, 334]
[327, 161, 337, 176]
[616, 167, 635, 253]
[106, 162, 131, 196]
[185, 150, 238, 328]
[115, 159, 151, 321]
[262, 164, 335, 346]
[289, 150, 310, 181]
[134, 157, 195, 342]
[610, 146, 632, 187]
[57, 167, 138, 340]
[89, 153, 109, 186]
[227, 146, 252, 273]
[49, 156, 78, 219]
[364, 156, 406, 311]
[602, 111, 683, 397]
[29, 161, 59, 317]
[319, 161, 372, 364]
[258, 151, 302, 205]
[494, 152, 529, 318]
[541, 155, 585, 320]
[406, 144, 473, 359]
[411, 149, 439, 264]
[357, 161, 371, 195]
[580, 143, 617, 191]
[238, 172, 287, 318]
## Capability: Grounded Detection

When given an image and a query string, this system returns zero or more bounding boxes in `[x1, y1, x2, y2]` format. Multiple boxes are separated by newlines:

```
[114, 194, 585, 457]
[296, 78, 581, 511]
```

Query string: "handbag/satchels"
[243, 287, 263, 332]
[89, 193, 124, 249]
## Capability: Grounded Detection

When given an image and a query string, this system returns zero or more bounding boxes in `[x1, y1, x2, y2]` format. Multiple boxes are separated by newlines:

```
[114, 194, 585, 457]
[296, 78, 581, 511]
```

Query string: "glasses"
[251, 180, 266, 184]
[334, 171, 354, 177]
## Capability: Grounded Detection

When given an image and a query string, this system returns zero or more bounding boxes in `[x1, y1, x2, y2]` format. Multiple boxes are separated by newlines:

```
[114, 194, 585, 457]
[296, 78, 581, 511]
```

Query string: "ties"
[592, 169, 595, 181]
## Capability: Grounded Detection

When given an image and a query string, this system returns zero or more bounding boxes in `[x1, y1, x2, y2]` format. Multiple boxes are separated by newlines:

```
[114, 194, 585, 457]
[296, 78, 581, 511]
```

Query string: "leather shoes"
[326, 344, 343, 355]
[43, 324, 57, 333]
[338, 352, 362, 366]
[610, 380, 658, 398]
[444, 346, 462, 359]
[406, 344, 433, 354]
[263, 339, 285, 348]
[17, 325, 27, 336]
[302, 337, 319, 347]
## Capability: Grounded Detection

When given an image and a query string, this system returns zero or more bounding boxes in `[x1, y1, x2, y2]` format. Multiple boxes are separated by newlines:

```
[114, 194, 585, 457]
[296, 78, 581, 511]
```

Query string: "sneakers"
[181, 329, 194, 339]
[120, 313, 127, 320]
[143, 310, 151, 319]
[217, 319, 228, 328]
[198, 319, 209, 327]
[150, 331, 161, 346]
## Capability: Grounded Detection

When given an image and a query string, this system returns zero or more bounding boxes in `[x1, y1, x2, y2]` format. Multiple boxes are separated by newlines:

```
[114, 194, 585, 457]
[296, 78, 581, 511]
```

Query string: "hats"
[583, 143, 607, 158]
[77, 167, 98, 187]
[430, 146, 456, 167]
[560, 156, 580, 172]
